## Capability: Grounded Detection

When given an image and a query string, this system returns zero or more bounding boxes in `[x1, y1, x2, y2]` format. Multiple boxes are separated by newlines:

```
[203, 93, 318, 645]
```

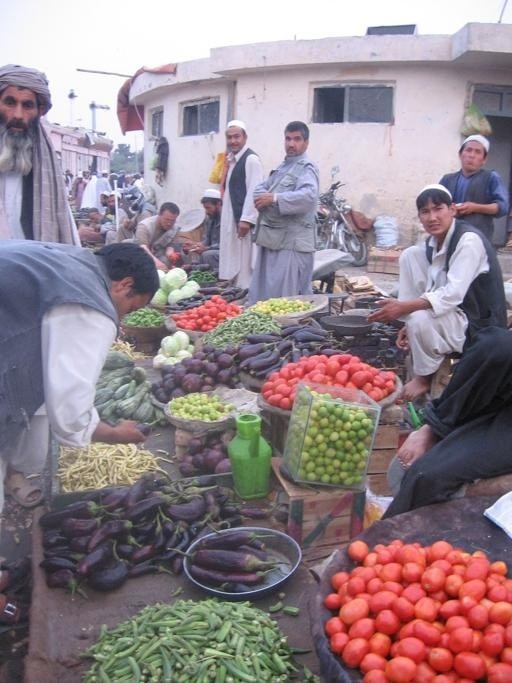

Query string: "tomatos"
[261, 353, 397, 411]
[171, 294, 244, 328]
[325, 539, 512, 683]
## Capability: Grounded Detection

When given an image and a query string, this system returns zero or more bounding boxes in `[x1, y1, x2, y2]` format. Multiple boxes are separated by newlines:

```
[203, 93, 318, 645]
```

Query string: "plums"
[286, 386, 373, 486]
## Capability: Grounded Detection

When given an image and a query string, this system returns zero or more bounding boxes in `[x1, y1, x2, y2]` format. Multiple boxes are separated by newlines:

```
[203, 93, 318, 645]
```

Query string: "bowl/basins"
[184, 527, 301, 600]
[320, 315, 374, 336]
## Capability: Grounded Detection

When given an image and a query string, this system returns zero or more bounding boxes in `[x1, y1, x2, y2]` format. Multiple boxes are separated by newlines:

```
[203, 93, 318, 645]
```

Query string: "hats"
[202, 189, 221, 199]
[416, 184, 452, 203]
[100, 191, 122, 199]
[227, 120, 245, 132]
[461, 134, 490, 154]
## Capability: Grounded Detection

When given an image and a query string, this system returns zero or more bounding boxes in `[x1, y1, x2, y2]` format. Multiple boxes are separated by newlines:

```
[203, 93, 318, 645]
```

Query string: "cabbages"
[149, 267, 199, 306]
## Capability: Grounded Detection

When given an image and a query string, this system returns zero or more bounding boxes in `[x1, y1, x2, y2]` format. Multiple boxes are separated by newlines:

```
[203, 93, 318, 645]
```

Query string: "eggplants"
[151, 322, 350, 403]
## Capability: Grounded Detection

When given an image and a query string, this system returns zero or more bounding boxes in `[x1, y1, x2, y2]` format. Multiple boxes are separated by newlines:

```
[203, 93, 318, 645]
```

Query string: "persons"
[0, 63, 82, 509]
[0, 239, 161, 627]
[368, 183, 508, 400]
[218, 120, 264, 287]
[64, 168, 182, 266]
[381, 326, 511, 517]
[439, 135, 510, 244]
[249, 121, 320, 303]
[189, 189, 222, 273]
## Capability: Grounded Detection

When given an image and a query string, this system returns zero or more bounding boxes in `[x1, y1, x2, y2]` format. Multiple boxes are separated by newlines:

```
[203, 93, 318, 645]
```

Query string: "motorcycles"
[314, 180, 368, 266]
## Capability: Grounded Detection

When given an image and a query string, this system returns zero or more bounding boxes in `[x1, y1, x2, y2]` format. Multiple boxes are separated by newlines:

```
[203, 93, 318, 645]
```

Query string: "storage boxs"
[281, 381, 381, 491]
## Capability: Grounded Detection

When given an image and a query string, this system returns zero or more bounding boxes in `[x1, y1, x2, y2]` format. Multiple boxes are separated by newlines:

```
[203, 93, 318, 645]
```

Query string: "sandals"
[4, 473, 45, 507]
[0, 556, 28, 624]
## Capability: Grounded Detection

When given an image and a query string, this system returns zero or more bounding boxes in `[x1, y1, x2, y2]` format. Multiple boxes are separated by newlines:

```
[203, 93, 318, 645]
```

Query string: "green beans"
[201, 309, 281, 347]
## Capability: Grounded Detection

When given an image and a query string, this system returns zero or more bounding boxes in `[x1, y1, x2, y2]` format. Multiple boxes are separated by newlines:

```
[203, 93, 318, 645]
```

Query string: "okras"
[80, 587, 315, 682]
